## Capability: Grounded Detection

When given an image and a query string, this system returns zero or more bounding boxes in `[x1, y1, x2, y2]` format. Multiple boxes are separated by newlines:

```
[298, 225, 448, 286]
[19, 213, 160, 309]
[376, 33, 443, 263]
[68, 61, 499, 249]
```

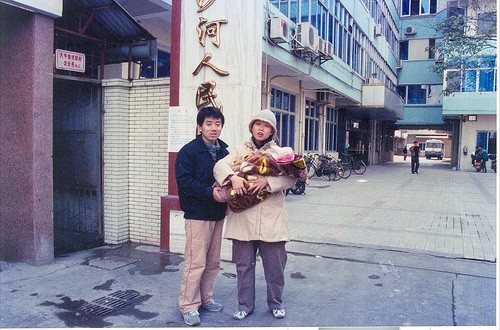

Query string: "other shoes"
[412, 171, 418, 174]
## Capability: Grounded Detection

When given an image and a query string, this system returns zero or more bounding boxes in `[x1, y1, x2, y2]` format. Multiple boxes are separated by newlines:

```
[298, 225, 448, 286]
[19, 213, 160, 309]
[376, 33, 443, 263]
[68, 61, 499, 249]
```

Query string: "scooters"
[471, 154, 486, 172]
[489, 155, 496, 173]
[285, 180, 309, 196]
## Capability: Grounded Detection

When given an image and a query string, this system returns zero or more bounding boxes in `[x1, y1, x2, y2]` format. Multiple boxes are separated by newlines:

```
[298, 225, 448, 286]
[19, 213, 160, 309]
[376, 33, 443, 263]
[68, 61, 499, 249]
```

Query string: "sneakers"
[202, 301, 223, 312]
[182, 310, 201, 326]
[233, 310, 248, 319]
[269, 309, 286, 319]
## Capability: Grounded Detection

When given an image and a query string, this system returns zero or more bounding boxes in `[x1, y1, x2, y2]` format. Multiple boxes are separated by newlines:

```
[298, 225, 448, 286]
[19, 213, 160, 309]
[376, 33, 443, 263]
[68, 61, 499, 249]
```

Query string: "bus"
[423, 139, 444, 160]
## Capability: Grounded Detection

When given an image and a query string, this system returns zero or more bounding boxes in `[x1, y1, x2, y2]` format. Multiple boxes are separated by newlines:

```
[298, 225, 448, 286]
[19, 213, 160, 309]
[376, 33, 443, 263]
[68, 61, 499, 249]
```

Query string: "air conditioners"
[404, 26, 417, 36]
[265, 16, 290, 46]
[290, 22, 333, 69]
[374, 24, 383, 38]
[365, 78, 374, 85]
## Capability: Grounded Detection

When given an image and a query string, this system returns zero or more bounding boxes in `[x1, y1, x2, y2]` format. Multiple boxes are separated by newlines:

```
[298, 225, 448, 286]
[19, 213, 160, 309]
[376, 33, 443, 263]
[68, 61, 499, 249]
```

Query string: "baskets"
[304, 155, 310, 163]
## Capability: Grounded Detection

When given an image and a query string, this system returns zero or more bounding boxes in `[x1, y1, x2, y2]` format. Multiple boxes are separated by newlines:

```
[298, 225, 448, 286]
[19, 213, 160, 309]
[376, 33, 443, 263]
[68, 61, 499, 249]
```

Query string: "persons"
[471, 145, 489, 173]
[402, 141, 421, 174]
[212, 109, 299, 319]
[174, 107, 228, 326]
[213, 151, 308, 212]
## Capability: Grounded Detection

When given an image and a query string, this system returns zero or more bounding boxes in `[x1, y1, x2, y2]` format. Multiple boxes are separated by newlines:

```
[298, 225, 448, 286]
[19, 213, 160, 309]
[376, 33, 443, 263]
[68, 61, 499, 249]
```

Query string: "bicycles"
[303, 151, 351, 182]
[335, 152, 367, 176]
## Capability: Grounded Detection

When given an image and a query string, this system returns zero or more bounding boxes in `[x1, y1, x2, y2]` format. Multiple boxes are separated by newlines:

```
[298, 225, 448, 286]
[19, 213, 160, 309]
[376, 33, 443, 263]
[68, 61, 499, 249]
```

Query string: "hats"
[249, 109, 277, 138]
